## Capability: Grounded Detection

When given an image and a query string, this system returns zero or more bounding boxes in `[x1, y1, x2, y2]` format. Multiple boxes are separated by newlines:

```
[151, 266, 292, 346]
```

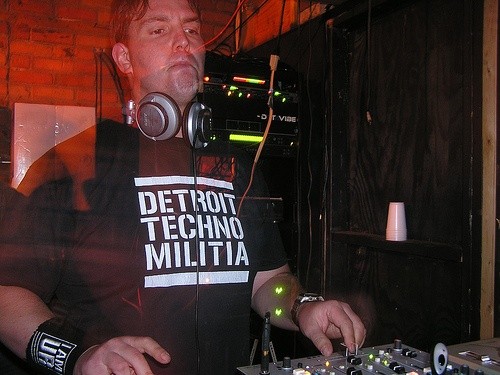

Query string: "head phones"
[126, 92, 217, 150]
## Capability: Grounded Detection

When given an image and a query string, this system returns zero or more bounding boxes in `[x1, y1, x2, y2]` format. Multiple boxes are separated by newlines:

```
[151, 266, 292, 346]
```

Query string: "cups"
[385, 202, 408, 241]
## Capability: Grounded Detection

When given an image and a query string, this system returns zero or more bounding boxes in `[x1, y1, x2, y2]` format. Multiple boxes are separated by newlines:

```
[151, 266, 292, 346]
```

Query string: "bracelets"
[26, 317, 99, 375]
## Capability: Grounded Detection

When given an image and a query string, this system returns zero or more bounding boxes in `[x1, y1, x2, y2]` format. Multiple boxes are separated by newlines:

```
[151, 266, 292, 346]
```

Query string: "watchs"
[291, 293, 324, 333]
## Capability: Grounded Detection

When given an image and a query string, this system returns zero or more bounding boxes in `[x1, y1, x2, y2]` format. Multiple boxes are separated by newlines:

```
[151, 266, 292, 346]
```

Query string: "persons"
[0, 180, 16, 218]
[0, 0, 366, 375]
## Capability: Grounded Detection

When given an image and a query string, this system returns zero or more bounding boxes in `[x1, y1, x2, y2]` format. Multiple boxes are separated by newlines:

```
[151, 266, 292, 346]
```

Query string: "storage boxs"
[9, 101, 97, 212]
[445, 337, 500, 375]
